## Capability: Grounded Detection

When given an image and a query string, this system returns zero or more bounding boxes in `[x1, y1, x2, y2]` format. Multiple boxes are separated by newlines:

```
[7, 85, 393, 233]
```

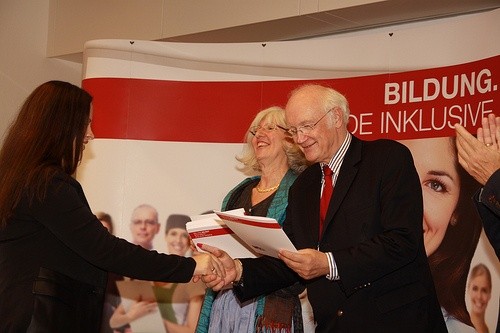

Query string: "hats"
[165, 215, 192, 234]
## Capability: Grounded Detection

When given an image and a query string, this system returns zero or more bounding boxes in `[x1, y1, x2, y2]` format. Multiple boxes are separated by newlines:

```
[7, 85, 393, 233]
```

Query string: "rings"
[485, 143, 493, 147]
[210, 266, 215, 271]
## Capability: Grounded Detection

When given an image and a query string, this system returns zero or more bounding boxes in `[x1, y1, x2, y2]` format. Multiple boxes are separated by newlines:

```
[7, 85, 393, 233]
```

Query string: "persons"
[190, 83, 449, 333]
[454, 113, 500, 264]
[95, 136, 492, 333]
[0, 80, 226, 333]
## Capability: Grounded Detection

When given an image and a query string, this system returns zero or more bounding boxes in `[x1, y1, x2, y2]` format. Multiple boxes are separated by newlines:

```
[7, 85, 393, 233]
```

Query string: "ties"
[319, 165, 333, 242]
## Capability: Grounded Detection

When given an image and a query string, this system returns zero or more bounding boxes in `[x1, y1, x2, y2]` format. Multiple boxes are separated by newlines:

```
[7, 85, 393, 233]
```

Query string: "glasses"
[284, 107, 335, 138]
[249, 123, 287, 135]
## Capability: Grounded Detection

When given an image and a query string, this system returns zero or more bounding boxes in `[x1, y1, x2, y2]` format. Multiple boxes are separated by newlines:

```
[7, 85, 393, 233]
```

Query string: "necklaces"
[256, 184, 279, 193]
